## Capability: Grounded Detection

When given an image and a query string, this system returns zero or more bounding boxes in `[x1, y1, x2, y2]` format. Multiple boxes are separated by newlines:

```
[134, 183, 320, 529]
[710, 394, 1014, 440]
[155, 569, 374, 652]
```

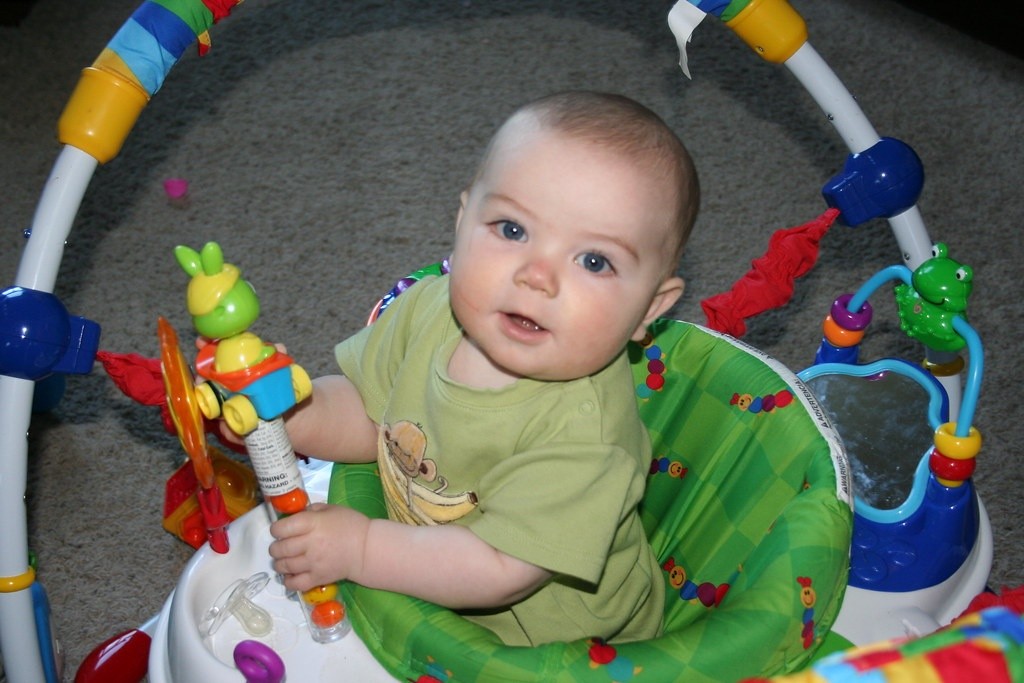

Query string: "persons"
[195, 91, 703, 647]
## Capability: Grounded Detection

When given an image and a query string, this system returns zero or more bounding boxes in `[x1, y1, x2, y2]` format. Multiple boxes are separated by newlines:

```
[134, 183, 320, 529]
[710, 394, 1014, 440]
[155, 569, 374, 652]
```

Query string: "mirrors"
[798, 369, 936, 513]
